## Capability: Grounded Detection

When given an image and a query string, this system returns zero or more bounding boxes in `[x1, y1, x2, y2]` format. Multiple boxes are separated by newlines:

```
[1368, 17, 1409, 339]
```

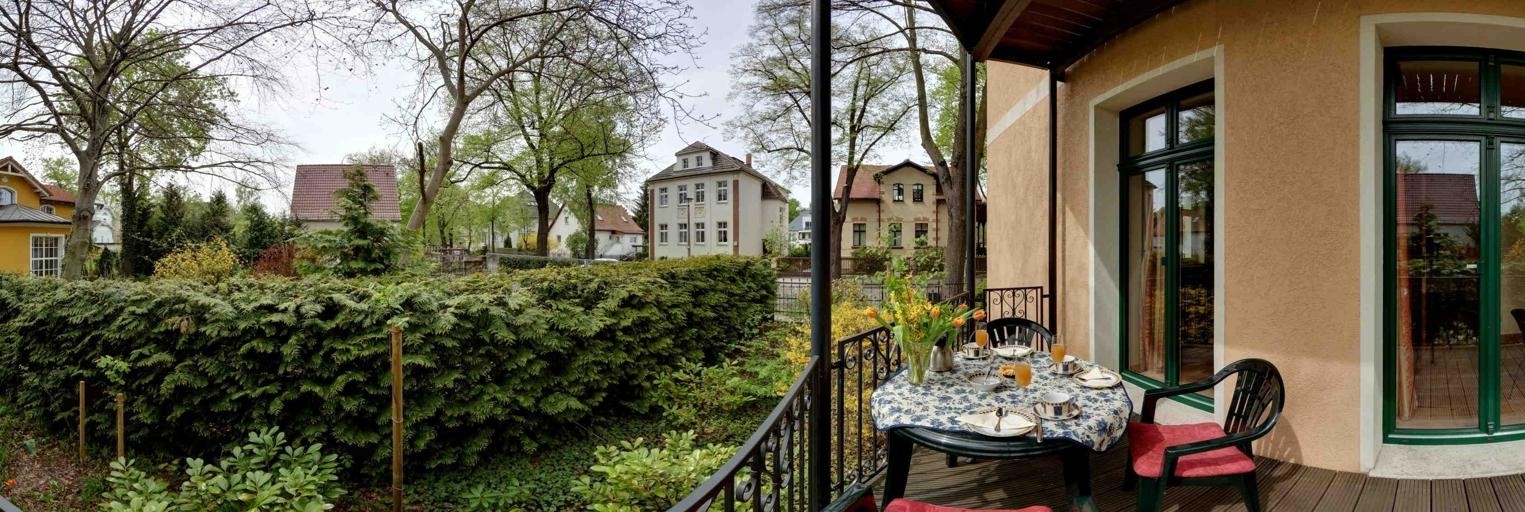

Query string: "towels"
[1076, 366, 1112, 388]
[955, 411, 1037, 430]
[990, 347, 1033, 356]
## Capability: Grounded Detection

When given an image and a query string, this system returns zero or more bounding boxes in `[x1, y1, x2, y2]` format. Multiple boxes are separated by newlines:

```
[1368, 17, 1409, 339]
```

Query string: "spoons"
[1076, 376, 1112, 384]
[994, 407, 1007, 432]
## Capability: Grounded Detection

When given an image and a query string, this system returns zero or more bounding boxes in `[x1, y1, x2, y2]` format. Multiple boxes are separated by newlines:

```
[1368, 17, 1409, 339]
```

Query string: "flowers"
[863, 269, 986, 382]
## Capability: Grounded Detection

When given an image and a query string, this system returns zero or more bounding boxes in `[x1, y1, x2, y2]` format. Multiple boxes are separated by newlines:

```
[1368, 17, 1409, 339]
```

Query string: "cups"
[1042, 391, 1077, 418]
[1058, 354, 1080, 373]
[961, 341, 984, 357]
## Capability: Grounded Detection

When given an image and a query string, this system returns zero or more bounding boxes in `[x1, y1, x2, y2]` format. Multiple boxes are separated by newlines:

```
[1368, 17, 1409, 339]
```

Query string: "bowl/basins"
[965, 370, 1004, 392]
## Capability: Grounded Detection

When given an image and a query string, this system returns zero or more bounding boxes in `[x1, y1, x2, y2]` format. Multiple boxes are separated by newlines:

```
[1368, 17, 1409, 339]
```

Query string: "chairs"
[1121, 358, 1285, 512]
[818, 483, 1099, 512]
[1508, 309, 1525, 400]
[968, 318, 1054, 353]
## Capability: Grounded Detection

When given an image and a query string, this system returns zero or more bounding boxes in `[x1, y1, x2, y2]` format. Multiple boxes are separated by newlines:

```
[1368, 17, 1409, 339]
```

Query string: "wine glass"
[974, 322, 988, 364]
[1013, 356, 1033, 400]
[1051, 334, 1066, 374]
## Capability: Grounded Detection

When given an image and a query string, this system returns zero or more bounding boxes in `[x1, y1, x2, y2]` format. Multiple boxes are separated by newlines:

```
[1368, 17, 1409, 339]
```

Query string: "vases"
[906, 343, 934, 385]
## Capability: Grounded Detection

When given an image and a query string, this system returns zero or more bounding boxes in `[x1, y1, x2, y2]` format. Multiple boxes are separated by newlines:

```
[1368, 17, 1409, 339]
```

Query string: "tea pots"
[929, 335, 952, 372]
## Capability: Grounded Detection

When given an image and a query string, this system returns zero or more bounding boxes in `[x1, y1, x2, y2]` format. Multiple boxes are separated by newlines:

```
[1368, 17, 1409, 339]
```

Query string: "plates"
[1049, 363, 1083, 376]
[967, 403, 1039, 439]
[958, 349, 991, 359]
[1032, 402, 1081, 421]
[1072, 367, 1122, 388]
[995, 344, 1032, 359]
